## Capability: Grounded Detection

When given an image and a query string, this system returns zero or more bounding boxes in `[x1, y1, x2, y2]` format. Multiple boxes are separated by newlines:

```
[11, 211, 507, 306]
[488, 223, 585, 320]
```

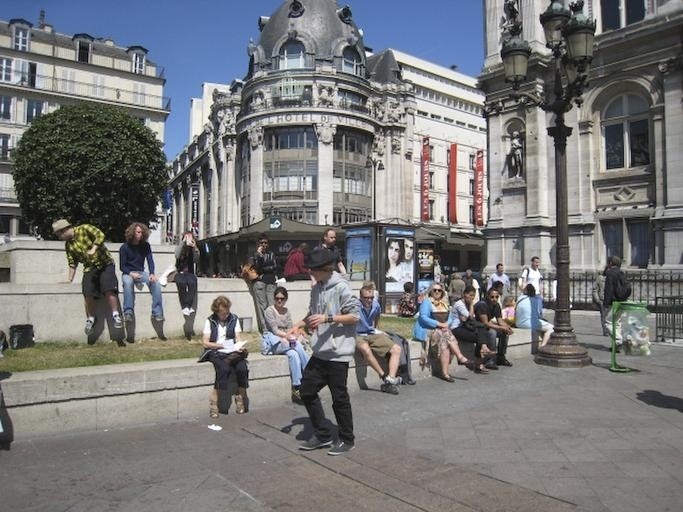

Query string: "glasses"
[274, 297, 287, 301]
[361, 296, 374, 300]
[489, 295, 499, 298]
[430, 288, 444, 293]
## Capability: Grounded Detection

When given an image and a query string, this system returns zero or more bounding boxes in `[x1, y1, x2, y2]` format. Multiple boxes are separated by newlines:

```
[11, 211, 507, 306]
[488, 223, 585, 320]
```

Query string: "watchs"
[327, 314, 334, 324]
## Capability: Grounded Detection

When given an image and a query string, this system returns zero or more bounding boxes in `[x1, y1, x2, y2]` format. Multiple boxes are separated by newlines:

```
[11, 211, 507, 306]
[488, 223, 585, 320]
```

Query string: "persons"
[593, 267, 612, 337]
[461, 270, 480, 293]
[450, 287, 497, 372]
[51, 220, 123, 331]
[487, 264, 512, 293]
[249, 235, 281, 336]
[515, 284, 557, 349]
[400, 281, 419, 317]
[353, 284, 402, 396]
[316, 229, 349, 280]
[204, 296, 248, 419]
[386, 238, 414, 282]
[173, 231, 198, 317]
[604, 255, 637, 351]
[264, 287, 309, 401]
[509, 129, 526, 177]
[385, 329, 417, 386]
[473, 288, 515, 369]
[118, 220, 166, 323]
[448, 269, 466, 304]
[521, 257, 544, 300]
[284, 249, 360, 455]
[285, 242, 319, 285]
[415, 284, 476, 382]
[503, 294, 518, 325]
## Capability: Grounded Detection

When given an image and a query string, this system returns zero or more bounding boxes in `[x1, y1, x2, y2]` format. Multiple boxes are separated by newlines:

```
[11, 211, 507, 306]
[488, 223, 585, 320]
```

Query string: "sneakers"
[234, 397, 245, 414]
[123, 314, 133, 324]
[209, 401, 218, 418]
[485, 362, 499, 370]
[292, 387, 302, 401]
[150, 312, 164, 322]
[84, 313, 97, 336]
[182, 307, 195, 317]
[327, 440, 355, 456]
[298, 435, 334, 451]
[381, 374, 398, 385]
[381, 384, 399, 394]
[110, 311, 122, 329]
[497, 359, 513, 367]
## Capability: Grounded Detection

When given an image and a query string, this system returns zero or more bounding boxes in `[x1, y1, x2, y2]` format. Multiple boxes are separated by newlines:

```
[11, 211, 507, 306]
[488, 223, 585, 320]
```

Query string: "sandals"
[440, 374, 455, 382]
[457, 358, 475, 366]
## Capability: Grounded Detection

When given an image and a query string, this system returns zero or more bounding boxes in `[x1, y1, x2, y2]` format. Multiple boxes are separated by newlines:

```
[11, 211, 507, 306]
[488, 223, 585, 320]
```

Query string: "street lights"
[501, 0, 597, 368]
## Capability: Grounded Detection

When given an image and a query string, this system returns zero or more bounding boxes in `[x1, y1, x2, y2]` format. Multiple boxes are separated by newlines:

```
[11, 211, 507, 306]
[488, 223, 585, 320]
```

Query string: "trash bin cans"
[611, 301, 652, 358]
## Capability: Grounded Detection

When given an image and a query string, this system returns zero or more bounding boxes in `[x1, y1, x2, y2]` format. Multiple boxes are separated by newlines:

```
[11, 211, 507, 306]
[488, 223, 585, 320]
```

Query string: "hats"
[302, 246, 339, 269]
[51, 219, 73, 235]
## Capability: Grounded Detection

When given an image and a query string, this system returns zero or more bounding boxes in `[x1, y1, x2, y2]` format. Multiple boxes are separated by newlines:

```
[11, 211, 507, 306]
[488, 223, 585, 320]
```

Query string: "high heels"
[480, 350, 498, 358]
[475, 363, 490, 373]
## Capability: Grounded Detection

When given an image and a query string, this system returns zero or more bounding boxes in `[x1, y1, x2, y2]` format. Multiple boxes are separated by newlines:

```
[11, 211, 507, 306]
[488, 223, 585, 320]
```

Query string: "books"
[217, 340, 248, 354]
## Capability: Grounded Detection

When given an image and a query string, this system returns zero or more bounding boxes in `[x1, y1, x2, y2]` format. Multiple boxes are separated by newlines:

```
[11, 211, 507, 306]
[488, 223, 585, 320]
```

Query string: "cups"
[289, 336, 296, 348]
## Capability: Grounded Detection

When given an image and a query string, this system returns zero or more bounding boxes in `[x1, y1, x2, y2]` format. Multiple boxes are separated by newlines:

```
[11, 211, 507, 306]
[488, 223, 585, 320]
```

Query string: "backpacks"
[612, 272, 632, 301]
